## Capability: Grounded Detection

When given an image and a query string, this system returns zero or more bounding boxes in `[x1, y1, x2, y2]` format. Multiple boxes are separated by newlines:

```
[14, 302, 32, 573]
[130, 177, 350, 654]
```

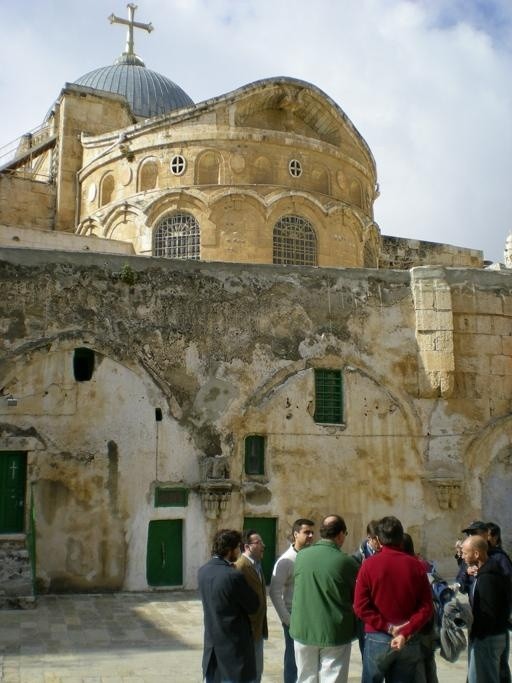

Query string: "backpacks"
[425, 567, 467, 661]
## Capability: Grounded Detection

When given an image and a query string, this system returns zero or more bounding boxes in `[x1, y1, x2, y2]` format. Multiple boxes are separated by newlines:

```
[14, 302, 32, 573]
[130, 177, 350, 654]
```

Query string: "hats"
[461, 521, 487, 532]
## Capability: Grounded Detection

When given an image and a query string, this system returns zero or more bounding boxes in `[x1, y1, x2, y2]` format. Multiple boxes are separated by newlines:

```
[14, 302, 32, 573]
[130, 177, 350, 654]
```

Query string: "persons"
[350, 517, 435, 683]
[350, 521, 512, 683]
[196, 529, 261, 682]
[234, 529, 270, 677]
[268, 518, 315, 682]
[288, 514, 359, 682]
